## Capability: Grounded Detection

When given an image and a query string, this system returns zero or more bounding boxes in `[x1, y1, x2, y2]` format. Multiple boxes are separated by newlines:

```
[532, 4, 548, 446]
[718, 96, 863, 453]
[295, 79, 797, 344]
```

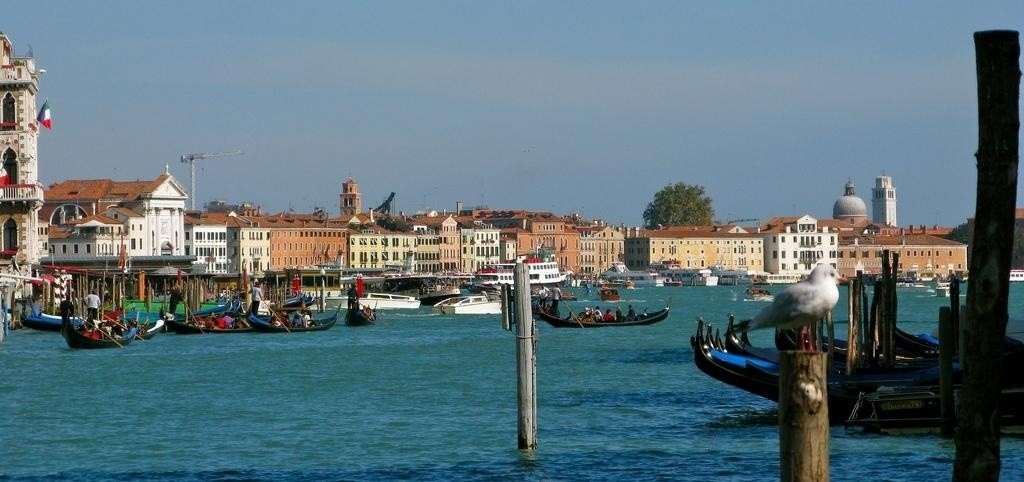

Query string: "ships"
[470, 244, 568, 294]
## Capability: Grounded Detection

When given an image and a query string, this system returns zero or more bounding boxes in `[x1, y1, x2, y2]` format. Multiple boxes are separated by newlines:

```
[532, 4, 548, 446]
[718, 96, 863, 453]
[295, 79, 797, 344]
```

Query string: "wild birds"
[723, 263, 849, 355]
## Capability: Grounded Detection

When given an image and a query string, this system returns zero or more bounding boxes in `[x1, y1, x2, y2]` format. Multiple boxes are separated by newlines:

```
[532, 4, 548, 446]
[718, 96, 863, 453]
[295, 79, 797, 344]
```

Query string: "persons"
[273, 310, 313, 327]
[585, 305, 635, 321]
[551, 282, 562, 316]
[98, 318, 127, 338]
[363, 305, 375, 320]
[169, 279, 182, 313]
[249, 281, 263, 318]
[539, 284, 550, 309]
[348, 283, 360, 309]
[84, 290, 101, 321]
[194, 312, 236, 328]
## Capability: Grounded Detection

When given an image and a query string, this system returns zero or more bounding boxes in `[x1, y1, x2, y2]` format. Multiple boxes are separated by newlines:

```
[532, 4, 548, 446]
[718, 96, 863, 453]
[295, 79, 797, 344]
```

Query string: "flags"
[37, 99, 52, 129]
[0, 160, 9, 188]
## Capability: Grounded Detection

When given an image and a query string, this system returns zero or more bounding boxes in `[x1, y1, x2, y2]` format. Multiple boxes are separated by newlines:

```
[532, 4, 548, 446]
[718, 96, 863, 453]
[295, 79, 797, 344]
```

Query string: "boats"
[322, 291, 421, 311]
[433, 295, 503, 316]
[935, 282, 951, 297]
[599, 289, 621, 301]
[344, 300, 378, 325]
[537, 304, 671, 328]
[57, 274, 343, 348]
[603, 263, 798, 287]
[383, 275, 461, 306]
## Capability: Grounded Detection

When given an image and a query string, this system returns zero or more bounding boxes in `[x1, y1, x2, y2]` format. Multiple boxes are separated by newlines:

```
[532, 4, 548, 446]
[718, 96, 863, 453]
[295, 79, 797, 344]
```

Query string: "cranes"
[180, 149, 244, 211]
